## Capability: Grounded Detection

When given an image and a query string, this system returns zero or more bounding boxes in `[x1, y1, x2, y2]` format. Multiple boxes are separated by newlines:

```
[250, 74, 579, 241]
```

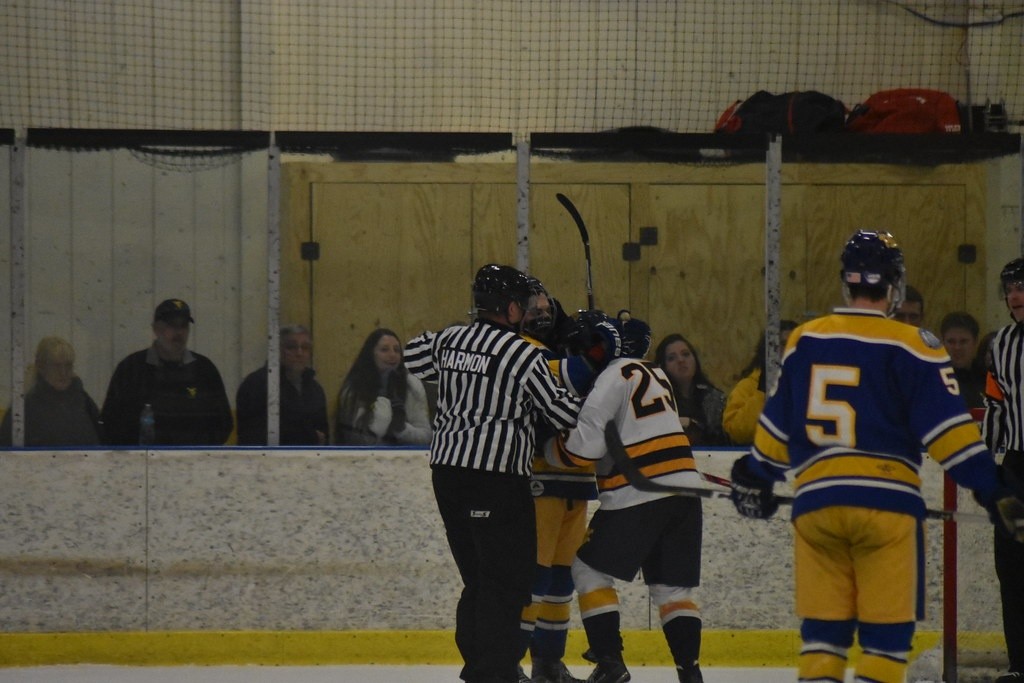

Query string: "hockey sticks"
[554, 190, 596, 312]
[604, 419, 1021, 530]
[699, 469, 731, 486]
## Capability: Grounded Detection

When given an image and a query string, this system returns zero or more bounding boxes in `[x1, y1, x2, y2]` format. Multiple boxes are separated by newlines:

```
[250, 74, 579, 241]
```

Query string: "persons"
[236, 323, 330, 447]
[404, 262, 713, 683]
[654, 333, 726, 446]
[1, 337, 101, 450]
[722, 320, 801, 445]
[101, 299, 233, 446]
[335, 328, 432, 447]
[892, 284, 999, 408]
[730, 229, 1024, 683]
[980, 257, 1024, 683]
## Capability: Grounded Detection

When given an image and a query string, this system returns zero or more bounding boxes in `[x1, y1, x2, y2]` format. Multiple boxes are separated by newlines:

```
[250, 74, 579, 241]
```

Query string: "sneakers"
[531, 660, 587, 683]
[676, 660, 703, 683]
[587, 664, 630, 683]
[516, 666, 531, 683]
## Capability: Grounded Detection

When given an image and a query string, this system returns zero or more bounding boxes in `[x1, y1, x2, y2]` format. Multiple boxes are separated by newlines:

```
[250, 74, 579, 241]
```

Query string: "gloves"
[731, 454, 779, 519]
[591, 318, 651, 360]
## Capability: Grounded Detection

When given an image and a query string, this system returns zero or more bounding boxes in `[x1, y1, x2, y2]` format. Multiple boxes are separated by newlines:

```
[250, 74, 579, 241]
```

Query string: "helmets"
[1000, 258, 1024, 281]
[554, 310, 607, 344]
[840, 229, 905, 286]
[472, 263, 528, 312]
[527, 277, 542, 287]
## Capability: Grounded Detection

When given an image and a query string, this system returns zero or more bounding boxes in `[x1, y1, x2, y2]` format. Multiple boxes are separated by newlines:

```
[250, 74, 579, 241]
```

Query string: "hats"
[154, 299, 195, 325]
